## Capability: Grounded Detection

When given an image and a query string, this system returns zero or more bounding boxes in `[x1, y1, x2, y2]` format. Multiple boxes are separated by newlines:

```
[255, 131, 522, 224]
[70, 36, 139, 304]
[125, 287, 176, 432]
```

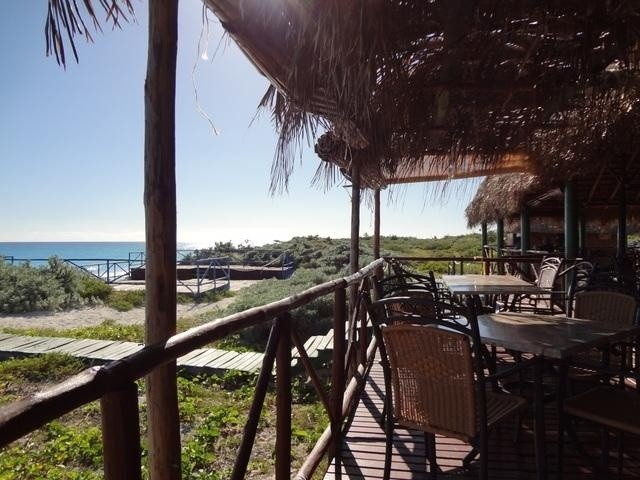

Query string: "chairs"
[359, 245, 640, 480]
